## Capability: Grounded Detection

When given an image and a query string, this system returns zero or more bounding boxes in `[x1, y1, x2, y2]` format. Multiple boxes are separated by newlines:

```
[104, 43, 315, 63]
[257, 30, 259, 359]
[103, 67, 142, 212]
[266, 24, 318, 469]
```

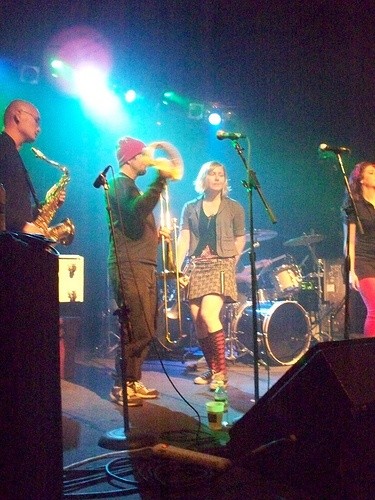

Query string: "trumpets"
[162, 255, 196, 319]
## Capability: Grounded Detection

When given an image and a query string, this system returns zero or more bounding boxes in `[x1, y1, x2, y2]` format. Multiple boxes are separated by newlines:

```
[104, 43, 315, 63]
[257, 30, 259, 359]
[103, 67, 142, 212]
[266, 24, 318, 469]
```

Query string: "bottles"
[214, 381, 228, 425]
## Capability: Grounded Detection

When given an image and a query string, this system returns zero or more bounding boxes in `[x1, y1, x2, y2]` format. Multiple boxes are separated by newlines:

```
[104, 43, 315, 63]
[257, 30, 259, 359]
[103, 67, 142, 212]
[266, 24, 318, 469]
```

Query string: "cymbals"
[242, 228, 279, 241]
[282, 233, 332, 248]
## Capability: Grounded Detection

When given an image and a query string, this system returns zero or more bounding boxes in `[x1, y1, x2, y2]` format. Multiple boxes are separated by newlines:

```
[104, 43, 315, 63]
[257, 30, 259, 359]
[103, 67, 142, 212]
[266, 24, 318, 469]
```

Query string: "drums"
[256, 288, 280, 302]
[229, 300, 312, 366]
[269, 262, 303, 298]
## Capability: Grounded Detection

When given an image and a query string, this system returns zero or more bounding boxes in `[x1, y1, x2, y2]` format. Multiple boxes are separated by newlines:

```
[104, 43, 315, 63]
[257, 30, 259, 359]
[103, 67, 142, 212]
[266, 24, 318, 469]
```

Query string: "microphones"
[94, 166, 109, 188]
[320, 144, 349, 152]
[216, 130, 247, 140]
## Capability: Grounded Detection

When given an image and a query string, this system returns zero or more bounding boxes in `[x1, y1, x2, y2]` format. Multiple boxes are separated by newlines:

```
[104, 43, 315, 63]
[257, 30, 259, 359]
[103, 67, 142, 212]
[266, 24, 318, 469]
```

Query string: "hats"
[117, 136, 145, 167]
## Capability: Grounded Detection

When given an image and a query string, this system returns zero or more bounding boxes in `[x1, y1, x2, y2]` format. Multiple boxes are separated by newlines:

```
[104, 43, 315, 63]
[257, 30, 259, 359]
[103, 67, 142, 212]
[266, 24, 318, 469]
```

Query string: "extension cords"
[152, 443, 231, 473]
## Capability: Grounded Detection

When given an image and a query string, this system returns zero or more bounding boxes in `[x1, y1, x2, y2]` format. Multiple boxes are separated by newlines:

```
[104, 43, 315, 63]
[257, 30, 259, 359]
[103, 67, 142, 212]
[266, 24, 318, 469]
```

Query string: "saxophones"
[31, 147, 75, 246]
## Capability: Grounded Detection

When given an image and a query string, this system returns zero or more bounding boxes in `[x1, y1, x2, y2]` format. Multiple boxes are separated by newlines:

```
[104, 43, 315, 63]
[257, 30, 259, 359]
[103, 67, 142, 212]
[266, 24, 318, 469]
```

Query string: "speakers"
[317, 258, 368, 335]
[228, 336, 375, 500]
[0, 229, 65, 500]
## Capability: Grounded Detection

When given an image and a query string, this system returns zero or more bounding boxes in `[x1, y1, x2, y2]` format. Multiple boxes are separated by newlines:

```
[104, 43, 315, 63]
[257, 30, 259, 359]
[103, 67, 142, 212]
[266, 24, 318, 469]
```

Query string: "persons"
[342, 161, 375, 337]
[0, 100, 66, 235]
[235, 257, 273, 297]
[103, 136, 174, 406]
[176, 161, 248, 390]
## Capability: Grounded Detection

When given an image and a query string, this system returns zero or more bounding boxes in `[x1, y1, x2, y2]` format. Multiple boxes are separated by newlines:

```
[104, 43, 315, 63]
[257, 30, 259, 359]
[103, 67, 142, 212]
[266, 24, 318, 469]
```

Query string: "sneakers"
[129, 380, 159, 399]
[210, 370, 228, 390]
[194, 369, 217, 384]
[109, 382, 144, 407]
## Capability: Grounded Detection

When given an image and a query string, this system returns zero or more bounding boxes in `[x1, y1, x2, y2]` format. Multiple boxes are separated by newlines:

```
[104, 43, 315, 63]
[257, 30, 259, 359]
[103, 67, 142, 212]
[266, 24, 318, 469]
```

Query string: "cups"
[205, 401, 225, 430]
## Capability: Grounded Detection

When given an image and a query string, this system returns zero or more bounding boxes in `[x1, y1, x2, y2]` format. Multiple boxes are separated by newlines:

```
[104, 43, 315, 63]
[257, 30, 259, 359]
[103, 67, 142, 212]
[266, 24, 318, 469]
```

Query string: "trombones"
[159, 181, 182, 344]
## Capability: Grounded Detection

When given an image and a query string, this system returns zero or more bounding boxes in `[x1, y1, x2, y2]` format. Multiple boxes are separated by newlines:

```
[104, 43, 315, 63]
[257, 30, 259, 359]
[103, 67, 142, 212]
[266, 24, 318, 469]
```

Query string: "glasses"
[18, 109, 40, 127]
